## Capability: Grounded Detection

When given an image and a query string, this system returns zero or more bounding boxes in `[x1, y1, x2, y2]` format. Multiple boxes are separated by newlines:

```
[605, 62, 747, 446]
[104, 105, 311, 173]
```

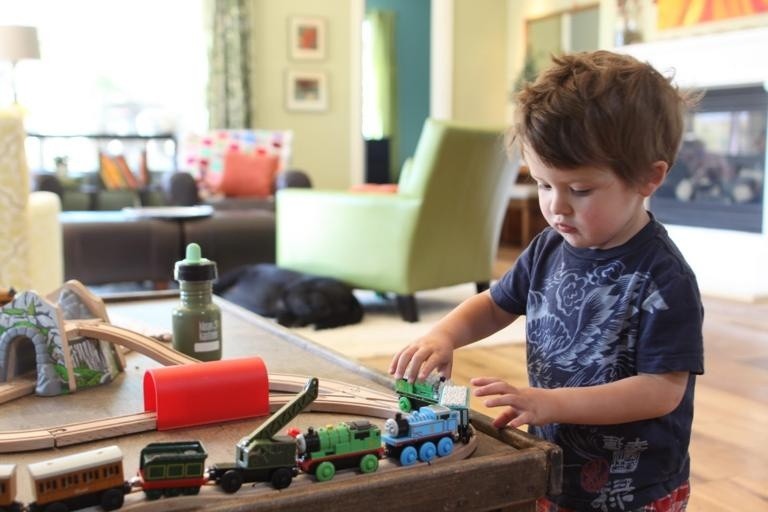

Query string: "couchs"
[33, 168, 310, 292]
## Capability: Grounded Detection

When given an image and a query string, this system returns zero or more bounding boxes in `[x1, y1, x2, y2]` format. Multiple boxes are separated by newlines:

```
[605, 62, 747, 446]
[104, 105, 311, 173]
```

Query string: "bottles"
[168, 244, 222, 360]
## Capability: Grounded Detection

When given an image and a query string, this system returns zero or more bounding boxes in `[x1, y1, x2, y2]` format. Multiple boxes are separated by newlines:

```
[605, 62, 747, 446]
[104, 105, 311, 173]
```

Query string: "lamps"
[4, 23, 40, 110]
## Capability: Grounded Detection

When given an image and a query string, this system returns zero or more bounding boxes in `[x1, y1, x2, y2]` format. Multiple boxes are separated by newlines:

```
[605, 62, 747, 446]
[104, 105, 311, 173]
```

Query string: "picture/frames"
[291, 18, 326, 59]
[283, 70, 326, 110]
[609, 0, 767, 53]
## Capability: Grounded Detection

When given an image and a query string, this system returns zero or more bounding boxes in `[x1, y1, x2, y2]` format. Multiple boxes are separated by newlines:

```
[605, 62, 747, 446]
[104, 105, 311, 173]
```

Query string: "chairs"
[273, 115, 524, 323]
[2, 116, 62, 304]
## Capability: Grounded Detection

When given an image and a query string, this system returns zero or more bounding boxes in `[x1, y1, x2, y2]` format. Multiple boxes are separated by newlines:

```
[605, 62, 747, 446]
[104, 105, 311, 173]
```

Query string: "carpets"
[292, 273, 528, 362]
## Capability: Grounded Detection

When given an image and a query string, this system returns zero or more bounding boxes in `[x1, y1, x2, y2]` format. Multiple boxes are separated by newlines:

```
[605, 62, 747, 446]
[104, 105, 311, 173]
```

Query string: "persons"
[388, 48, 705, 512]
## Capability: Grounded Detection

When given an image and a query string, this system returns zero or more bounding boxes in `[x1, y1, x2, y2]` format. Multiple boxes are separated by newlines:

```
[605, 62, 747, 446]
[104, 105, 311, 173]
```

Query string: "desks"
[0, 291, 564, 512]
